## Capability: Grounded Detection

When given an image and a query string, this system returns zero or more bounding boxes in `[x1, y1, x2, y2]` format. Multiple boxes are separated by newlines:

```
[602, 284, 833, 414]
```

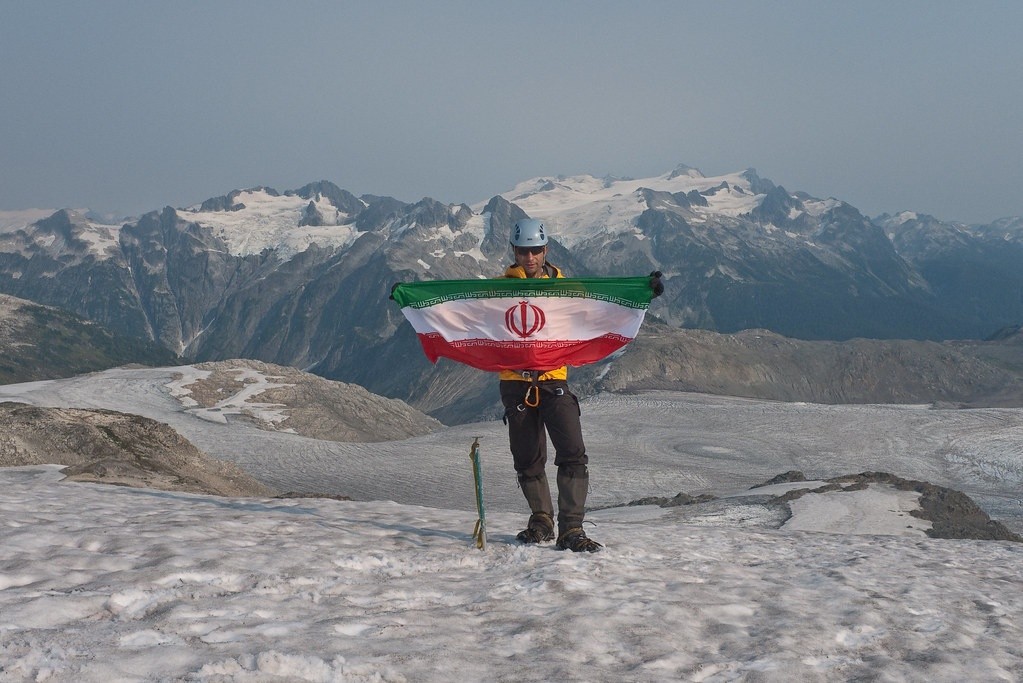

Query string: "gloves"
[389, 282, 402, 299]
[650, 271, 664, 298]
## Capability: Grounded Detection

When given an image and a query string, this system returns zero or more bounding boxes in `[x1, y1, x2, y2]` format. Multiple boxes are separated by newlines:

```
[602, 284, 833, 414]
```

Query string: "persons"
[389, 219, 664, 554]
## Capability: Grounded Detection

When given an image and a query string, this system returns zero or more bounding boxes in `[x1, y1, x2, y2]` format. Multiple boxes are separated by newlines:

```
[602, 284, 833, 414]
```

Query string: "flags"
[391, 276, 655, 370]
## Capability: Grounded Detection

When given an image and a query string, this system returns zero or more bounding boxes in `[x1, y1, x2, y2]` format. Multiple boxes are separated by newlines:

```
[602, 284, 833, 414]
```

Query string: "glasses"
[516, 246, 543, 255]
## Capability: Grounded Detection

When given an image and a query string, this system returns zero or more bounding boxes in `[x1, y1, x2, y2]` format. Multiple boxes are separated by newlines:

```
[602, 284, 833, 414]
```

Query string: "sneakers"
[516, 512, 554, 543]
[555, 528, 604, 553]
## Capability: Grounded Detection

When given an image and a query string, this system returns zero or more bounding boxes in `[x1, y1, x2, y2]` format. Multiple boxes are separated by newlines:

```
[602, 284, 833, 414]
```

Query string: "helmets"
[509, 219, 549, 247]
